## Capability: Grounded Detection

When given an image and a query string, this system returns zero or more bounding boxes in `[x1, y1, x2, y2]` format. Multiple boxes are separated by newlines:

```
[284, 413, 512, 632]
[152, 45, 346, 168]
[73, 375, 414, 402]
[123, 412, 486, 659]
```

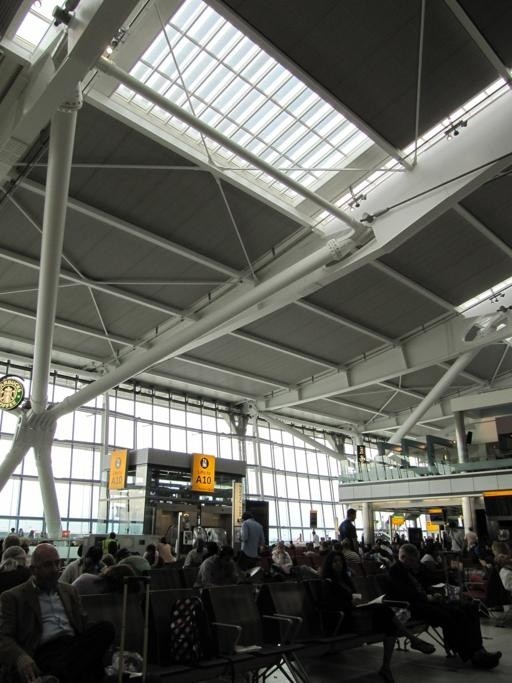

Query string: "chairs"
[76, 570, 445, 682]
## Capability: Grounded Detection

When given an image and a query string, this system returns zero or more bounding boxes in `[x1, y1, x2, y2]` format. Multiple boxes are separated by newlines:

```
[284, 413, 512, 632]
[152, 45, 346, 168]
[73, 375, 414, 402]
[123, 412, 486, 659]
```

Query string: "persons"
[239, 511, 265, 577]
[385, 544, 502, 671]
[269, 532, 362, 581]
[479, 540, 507, 611]
[2, 543, 116, 679]
[182, 538, 249, 594]
[2, 528, 48, 592]
[360, 523, 478, 577]
[339, 508, 359, 548]
[58, 532, 177, 595]
[313, 551, 436, 678]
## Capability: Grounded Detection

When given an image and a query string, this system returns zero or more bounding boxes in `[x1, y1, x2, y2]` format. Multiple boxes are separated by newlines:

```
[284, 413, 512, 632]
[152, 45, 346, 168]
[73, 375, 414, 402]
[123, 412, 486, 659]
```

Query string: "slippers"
[170, 586, 216, 662]
